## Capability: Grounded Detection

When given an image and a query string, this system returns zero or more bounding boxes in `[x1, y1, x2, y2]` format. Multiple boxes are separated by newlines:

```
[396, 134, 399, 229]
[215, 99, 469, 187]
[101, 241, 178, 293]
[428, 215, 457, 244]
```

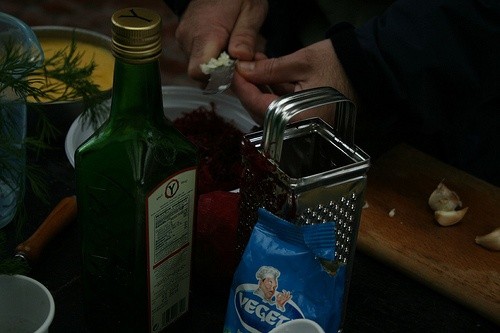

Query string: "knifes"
[203, 57, 234, 94]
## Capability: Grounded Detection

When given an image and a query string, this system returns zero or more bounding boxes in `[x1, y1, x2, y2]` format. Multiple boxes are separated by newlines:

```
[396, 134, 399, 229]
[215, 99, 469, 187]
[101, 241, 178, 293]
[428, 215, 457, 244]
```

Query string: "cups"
[0, 274, 54, 333]
[0, 12, 42, 226]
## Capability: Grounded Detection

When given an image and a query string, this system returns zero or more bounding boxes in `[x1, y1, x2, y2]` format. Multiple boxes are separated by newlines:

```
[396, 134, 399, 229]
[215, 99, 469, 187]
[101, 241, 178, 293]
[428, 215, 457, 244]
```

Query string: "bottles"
[75, 7, 194, 333]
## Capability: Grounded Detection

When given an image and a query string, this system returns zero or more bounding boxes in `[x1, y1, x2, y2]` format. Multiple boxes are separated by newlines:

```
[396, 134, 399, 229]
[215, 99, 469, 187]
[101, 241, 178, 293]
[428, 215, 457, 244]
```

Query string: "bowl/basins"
[66, 83, 262, 191]
[0, 25, 116, 139]
